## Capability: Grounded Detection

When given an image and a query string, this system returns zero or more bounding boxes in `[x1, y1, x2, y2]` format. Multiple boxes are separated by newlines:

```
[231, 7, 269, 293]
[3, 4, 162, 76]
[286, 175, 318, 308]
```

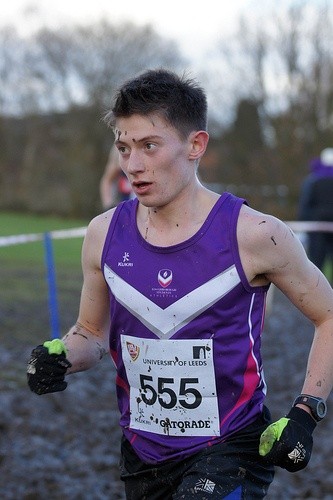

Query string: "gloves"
[27, 339, 72, 396]
[259, 406, 317, 473]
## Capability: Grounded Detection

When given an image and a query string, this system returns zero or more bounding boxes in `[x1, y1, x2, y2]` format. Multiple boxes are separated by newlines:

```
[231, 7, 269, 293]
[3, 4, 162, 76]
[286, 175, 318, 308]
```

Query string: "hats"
[321, 148, 333, 167]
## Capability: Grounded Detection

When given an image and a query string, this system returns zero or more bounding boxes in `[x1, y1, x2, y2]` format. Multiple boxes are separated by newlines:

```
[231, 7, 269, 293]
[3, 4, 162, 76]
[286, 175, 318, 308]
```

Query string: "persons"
[25, 70, 333, 499]
[95, 140, 137, 210]
[291, 146, 333, 278]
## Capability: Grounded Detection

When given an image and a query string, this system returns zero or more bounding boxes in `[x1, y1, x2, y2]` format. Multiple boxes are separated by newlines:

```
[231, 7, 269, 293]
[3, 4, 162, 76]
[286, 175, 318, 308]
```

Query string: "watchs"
[291, 393, 327, 422]
